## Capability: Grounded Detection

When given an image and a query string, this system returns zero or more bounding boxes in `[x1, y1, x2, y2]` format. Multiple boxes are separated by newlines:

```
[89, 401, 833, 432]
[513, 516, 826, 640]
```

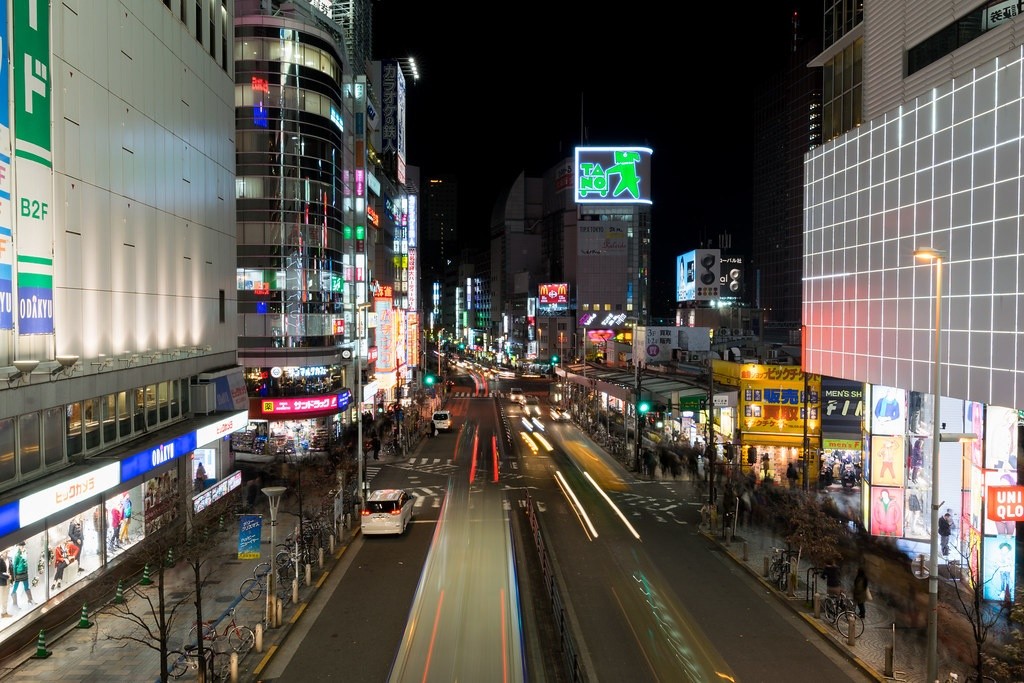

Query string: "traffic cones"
[137, 562, 154, 586]
[200, 514, 226, 540]
[185, 536, 193, 549]
[74, 601, 94, 629]
[31, 630, 53, 660]
[163, 547, 176, 568]
[109, 579, 127, 604]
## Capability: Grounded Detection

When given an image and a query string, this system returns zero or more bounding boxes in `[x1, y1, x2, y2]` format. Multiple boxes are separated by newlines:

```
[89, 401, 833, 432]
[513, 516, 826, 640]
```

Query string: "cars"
[361, 489, 415, 535]
[509, 387, 571, 424]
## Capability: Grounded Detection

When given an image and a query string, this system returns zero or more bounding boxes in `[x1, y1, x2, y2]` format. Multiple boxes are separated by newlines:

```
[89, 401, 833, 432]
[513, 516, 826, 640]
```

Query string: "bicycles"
[163, 471, 358, 679]
[767, 545, 865, 640]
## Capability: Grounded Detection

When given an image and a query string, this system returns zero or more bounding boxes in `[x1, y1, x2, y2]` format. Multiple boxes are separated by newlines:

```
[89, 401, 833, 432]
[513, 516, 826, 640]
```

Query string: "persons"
[852, 567, 867, 620]
[196, 462, 206, 494]
[600, 408, 863, 531]
[968, 401, 1016, 604]
[324, 400, 437, 475]
[66, 404, 73, 433]
[822, 558, 842, 618]
[863, 385, 901, 537]
[938, 513, 953, 557]
[907, 386, 928, 535]
[0, 491, 134, 618]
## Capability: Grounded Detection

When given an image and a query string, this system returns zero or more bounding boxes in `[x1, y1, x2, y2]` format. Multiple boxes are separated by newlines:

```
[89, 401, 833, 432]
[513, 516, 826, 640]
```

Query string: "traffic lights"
[637, 402, 668, 416]
[421, 376, 444, 384]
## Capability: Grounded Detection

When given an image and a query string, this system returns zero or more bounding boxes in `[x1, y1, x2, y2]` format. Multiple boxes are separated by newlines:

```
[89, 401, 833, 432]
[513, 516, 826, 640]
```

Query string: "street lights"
[435, 328, 444, 375]
[261, 486, 287, 628]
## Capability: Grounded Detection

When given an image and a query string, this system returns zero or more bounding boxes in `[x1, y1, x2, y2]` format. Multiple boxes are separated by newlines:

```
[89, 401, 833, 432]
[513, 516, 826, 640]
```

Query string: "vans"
[431, 410, 452, 431]
